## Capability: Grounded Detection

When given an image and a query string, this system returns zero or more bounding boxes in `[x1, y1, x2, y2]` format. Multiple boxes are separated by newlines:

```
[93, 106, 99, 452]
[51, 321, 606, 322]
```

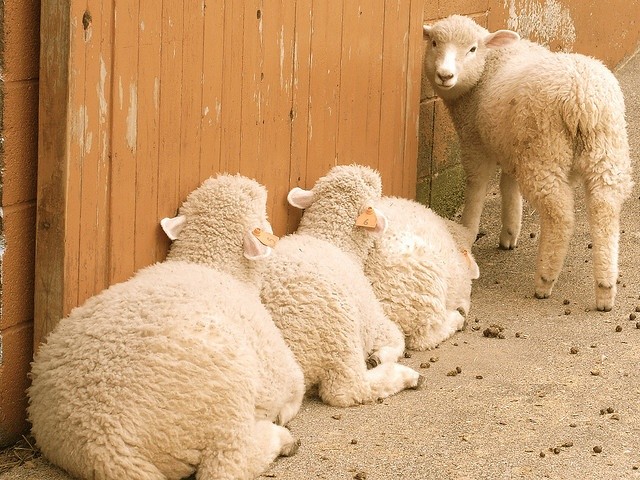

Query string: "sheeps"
[260, 166, 423, 406]
[27, 172, 304, 480]
[365, 194, 478, 351]
[421, 14, 634, 314]
